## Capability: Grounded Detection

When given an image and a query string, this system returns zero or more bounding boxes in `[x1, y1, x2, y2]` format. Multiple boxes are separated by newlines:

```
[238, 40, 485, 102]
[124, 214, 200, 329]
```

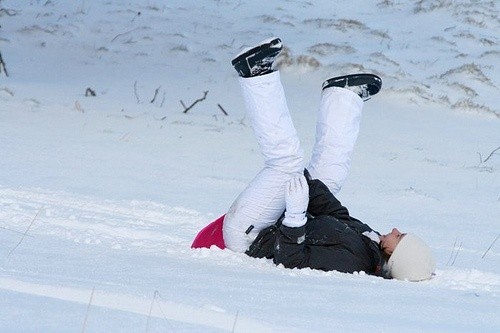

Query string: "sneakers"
[322, 75, 382, 102]
[231, 37, 283, 78]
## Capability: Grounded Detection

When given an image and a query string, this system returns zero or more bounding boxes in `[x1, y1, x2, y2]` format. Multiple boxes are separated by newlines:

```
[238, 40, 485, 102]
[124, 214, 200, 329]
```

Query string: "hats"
[387, 232, 436, 281]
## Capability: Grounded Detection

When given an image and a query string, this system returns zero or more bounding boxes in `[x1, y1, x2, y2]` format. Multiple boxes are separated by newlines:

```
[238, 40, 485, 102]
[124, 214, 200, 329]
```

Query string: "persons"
[222, 36, 436, 281]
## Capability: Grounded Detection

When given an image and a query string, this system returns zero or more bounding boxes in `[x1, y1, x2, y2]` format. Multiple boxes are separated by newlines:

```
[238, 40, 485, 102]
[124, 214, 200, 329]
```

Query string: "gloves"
[282, 175, 309, 222]
